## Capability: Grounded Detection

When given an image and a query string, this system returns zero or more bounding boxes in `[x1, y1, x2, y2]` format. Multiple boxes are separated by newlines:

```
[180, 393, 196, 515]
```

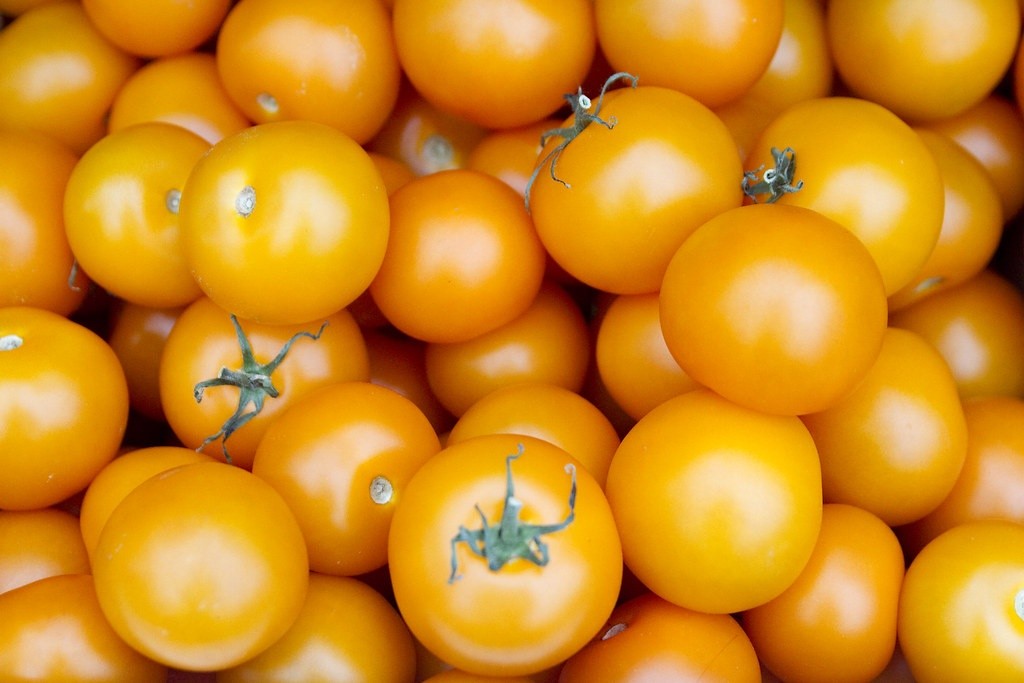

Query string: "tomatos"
[0, 0, 1024, 683]
